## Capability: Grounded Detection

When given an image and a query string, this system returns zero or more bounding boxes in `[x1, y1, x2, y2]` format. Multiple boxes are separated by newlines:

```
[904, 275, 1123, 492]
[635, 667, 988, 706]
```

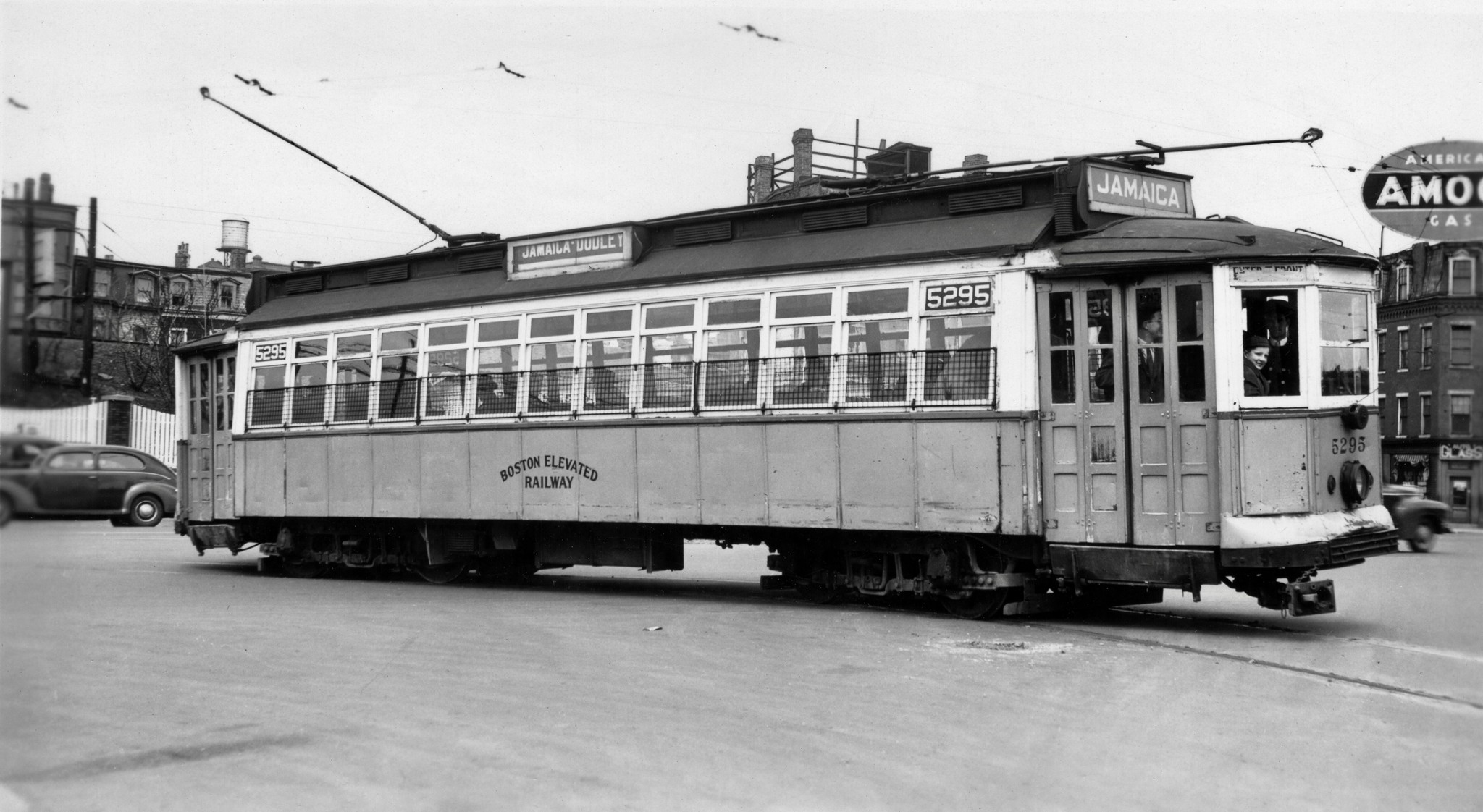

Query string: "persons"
[793, 357, 830, 403]
[1095, 307, 1164, 403]
[591, 370, 624, 406]
[941, 334, 990, 400]
[1244, 335, 1269, 396]
[475, 374, 552, 414]
[1260, 300, 1299, 396]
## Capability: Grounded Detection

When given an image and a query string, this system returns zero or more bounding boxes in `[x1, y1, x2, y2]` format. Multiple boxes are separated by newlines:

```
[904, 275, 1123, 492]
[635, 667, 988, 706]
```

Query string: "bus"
[173, 88, 1400, 619]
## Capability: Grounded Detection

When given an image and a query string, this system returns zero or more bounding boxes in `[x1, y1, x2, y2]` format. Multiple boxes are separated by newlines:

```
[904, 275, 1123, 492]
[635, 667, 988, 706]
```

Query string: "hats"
[1263, 298, 1291, 314]
[1243, 335, 1271, 348]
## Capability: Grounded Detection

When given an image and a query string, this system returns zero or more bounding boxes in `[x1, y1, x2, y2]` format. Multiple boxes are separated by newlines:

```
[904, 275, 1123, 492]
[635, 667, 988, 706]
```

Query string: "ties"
[1275, 341, 1282, 347]
[1146, 345, 1155, 377]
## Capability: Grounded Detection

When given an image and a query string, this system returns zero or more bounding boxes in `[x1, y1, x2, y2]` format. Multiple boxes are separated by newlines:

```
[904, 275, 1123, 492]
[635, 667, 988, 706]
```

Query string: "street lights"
[1, 202, 36, 392]
[54, 227, 97, 398]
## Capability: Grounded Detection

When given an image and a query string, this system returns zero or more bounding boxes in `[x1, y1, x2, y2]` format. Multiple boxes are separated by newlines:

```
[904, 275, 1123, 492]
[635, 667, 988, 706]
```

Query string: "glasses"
[1147, 318, 1163, 325]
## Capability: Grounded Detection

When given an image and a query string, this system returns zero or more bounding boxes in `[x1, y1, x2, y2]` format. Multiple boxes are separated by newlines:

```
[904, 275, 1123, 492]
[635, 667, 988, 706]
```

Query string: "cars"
[0, 444, 177, 527]
[1384, 483, 1455, 554]
[0, 433, 85, 470]
